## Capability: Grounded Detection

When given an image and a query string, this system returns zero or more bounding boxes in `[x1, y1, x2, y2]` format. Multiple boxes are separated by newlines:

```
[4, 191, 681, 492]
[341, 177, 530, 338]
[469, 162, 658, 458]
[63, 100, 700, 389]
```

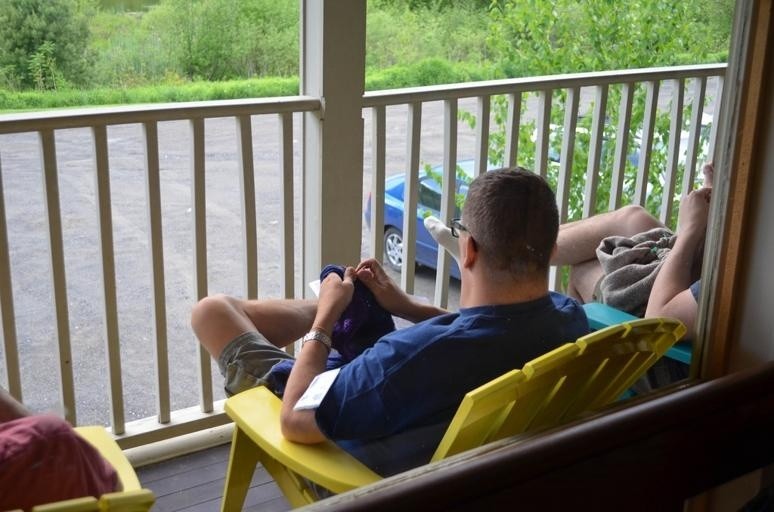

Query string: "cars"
[517, 110, 712, 209]
[365, 156, 502, 281]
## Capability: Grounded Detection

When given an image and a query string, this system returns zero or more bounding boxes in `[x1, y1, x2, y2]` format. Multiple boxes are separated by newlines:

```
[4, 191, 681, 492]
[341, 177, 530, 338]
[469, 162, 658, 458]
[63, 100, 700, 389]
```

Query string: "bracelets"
[304, 332, 331, 348]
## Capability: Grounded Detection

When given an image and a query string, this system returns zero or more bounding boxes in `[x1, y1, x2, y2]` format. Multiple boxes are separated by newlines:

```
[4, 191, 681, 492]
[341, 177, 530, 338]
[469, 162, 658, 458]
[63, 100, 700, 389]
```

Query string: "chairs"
[33, 423, 156, 512]
[220, 317, 692, 512]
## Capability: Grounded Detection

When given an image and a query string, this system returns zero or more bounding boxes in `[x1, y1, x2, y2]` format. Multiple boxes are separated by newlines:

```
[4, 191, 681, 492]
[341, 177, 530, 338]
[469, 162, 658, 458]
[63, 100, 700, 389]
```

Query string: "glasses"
[450, 218, 478, 252]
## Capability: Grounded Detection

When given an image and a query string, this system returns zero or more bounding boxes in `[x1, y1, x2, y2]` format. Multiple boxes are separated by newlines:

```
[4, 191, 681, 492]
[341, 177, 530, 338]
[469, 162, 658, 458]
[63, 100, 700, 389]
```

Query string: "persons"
[190, 166, 588, 499]
[423, 160, 714, 385]
[0, 385, 117, 512]
